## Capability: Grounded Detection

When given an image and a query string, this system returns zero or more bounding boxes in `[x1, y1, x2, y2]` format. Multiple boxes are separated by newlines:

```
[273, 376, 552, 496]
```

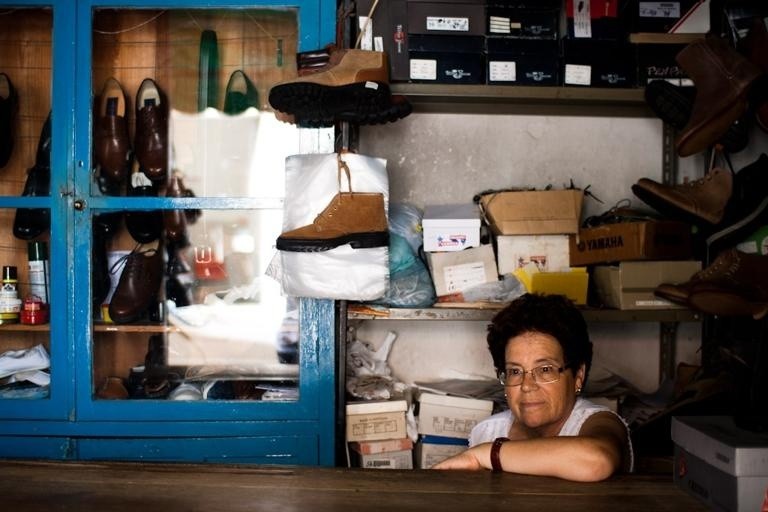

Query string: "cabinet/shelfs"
[0, 0, 341, 469]
[341, 79, 700, 389]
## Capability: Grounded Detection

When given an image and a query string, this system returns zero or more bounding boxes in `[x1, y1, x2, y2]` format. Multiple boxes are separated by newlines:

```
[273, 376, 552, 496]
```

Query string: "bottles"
[27, 240, 50, 305]
[0, 265, 22, 324]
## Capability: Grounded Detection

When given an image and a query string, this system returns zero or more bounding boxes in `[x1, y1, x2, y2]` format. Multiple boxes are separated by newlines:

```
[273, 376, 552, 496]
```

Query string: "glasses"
[495, 363, 570, 386]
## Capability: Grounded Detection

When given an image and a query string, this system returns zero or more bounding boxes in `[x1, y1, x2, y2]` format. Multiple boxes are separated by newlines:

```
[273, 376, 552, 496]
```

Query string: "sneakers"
[632, 151, 767, 246]
[653, 247, 768, 318]
[223, 69, 258, 115]
[0, 343, 51, 400]
[268, 46, 412, 128]
[0, 73, 16, 169]
[94, 334, 298, 401]
[277, 191, 390, 251]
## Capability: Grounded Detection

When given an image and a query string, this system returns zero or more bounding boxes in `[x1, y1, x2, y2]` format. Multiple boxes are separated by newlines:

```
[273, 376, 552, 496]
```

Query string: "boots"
[643, 31, 765, 155]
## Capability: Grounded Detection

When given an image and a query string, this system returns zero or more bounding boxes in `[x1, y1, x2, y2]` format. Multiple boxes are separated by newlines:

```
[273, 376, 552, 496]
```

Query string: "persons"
[430, 287, 634, 482]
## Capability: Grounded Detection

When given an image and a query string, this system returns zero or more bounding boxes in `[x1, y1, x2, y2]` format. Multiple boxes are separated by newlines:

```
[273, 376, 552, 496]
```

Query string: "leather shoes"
[94, 77, 185, 324]
[13, 111, 50, 240]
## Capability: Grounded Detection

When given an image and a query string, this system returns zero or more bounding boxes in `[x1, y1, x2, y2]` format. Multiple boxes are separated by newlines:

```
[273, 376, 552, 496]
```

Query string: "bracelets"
[490, 435, 510, 470]
